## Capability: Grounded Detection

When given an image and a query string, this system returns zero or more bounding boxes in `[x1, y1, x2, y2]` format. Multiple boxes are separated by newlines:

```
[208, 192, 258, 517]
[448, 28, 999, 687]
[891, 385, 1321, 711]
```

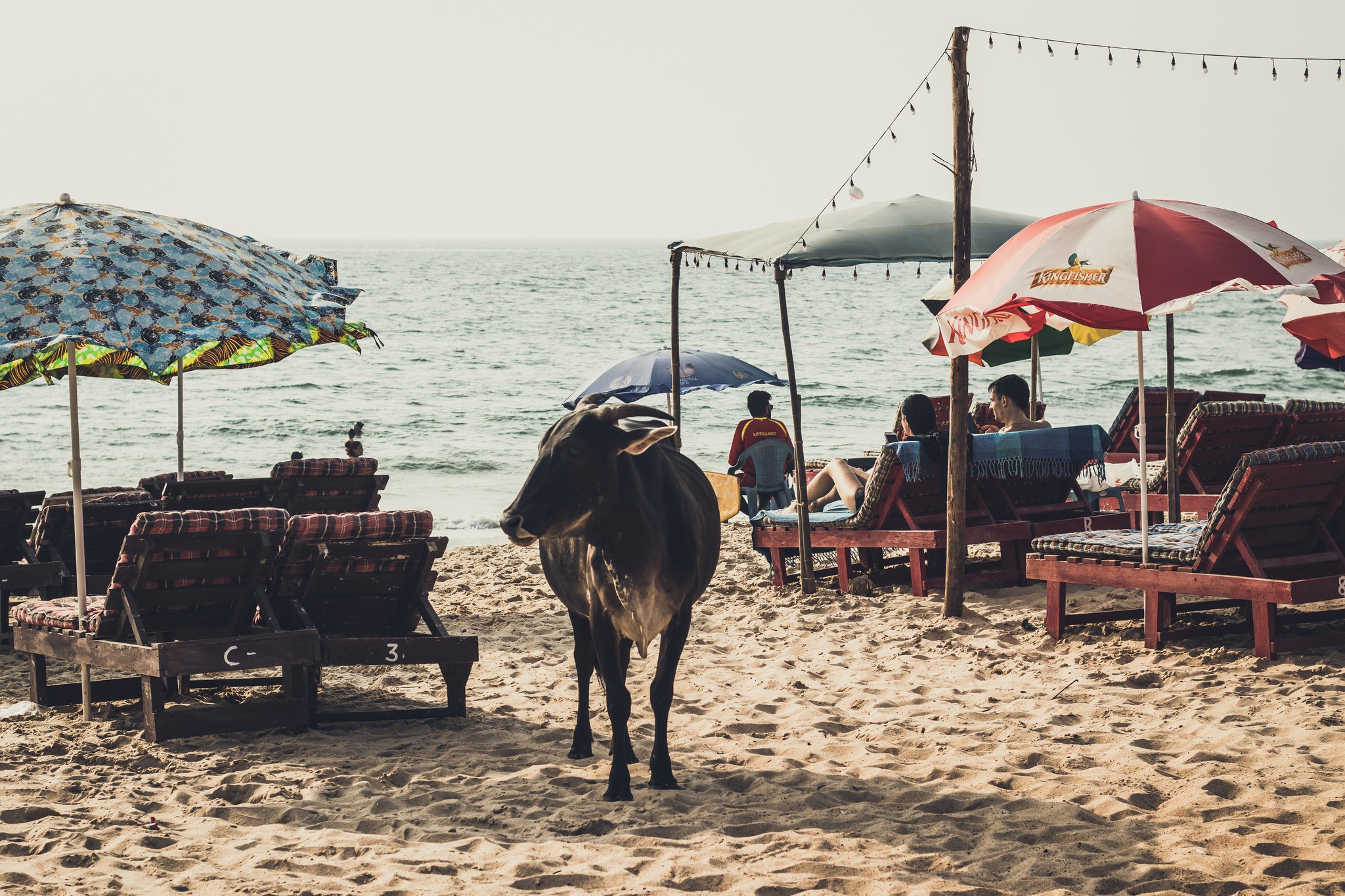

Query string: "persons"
[345, 440, 364, 458]
[726, 388, 797, 518]
[773, 391, 937, 516]
[975, 375, 1051, 435]
[291, 450, 304, 460]
[347, 428, 355, 441]
[353, 421, 365, 435]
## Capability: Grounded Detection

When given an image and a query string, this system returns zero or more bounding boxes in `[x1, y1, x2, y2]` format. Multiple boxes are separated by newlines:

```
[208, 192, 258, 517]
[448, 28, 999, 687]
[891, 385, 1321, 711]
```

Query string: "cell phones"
[884, 432, 898, 443]
[966, 412, 978, 434]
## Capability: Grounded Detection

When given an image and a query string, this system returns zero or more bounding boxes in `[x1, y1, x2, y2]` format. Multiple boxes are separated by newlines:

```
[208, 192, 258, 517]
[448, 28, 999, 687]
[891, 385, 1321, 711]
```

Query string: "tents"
[665, 190, 1185, 594]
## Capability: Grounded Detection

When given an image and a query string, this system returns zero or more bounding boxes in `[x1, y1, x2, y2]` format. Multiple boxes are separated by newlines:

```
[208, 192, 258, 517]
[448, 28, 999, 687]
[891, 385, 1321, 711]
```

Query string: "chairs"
[0, 457, 393, 638]
[869, 423, 1132, 588]
[1097, 400, 1290, 530]
[1025, 440, 1344, 662]
[1271, 398, 1345, 449]
[178, 509, 482, 729]
[11, 505, 318, 744]
[1063, 386, 1199, 515]
[752, 432, 1031, 598]
[726, 438, 796, 524]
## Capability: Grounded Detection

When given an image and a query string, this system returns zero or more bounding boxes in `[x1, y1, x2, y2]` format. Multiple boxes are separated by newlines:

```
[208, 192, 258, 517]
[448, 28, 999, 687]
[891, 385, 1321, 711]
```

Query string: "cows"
[499, 405, 720, 801]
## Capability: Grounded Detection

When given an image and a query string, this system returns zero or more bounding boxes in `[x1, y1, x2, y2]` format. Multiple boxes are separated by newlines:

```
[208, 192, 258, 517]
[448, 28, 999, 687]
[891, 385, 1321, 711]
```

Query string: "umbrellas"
[562, 345, 791, 432]
[0, 194, 368, 722]
[0, 321, 388, 483]
[932, 190, 1345, 634]
[1272, 239, 1345, 372]
